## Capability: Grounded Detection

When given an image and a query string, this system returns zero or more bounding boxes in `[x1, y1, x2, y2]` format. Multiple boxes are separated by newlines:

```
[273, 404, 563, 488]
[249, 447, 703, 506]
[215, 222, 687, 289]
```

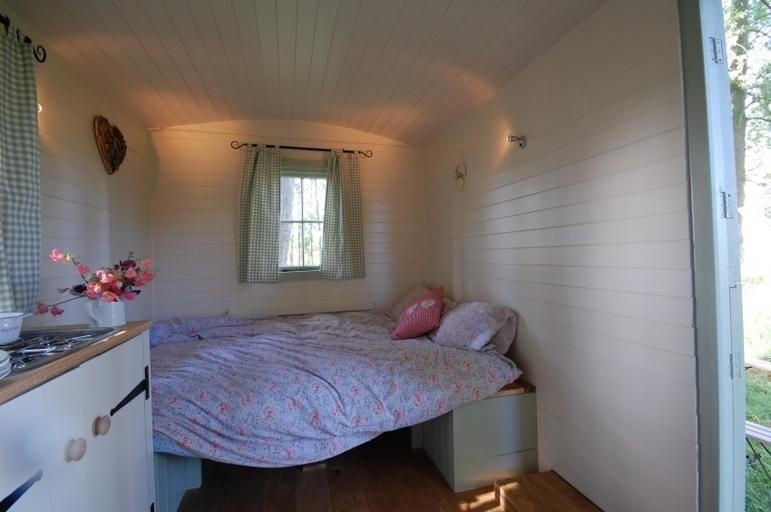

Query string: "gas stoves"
[0, 328, 116, 374]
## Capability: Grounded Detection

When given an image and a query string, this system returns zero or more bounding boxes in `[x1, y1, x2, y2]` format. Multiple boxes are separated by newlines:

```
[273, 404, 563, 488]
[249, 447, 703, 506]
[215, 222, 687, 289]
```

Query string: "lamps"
[506, 135, 526, 151]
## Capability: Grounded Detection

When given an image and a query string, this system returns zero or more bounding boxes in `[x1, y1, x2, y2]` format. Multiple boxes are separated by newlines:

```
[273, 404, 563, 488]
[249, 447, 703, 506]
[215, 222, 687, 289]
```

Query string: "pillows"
[388, 283, 520, 358]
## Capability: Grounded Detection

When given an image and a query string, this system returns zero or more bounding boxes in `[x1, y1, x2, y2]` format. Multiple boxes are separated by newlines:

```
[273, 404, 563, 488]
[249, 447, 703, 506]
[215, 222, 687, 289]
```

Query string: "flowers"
[36, 245, 155, 319]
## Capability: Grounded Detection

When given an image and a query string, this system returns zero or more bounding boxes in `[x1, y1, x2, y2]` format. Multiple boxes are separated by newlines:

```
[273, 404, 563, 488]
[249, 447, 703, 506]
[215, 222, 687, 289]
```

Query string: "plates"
[0, 350, 12, 383]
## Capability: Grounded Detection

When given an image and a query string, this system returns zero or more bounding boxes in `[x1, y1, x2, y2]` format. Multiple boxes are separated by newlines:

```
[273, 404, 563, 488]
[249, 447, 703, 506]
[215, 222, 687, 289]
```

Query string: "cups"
[0, 312, 24, 344]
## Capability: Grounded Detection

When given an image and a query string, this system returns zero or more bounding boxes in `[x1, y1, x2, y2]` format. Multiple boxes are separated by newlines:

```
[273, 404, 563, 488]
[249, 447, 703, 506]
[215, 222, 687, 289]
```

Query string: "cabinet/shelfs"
[0, 328, 147, 511]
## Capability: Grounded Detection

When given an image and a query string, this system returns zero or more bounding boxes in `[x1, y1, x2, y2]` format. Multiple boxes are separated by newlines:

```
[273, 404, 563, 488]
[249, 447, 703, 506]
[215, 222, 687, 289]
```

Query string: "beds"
[149, 306, 543, 512]
